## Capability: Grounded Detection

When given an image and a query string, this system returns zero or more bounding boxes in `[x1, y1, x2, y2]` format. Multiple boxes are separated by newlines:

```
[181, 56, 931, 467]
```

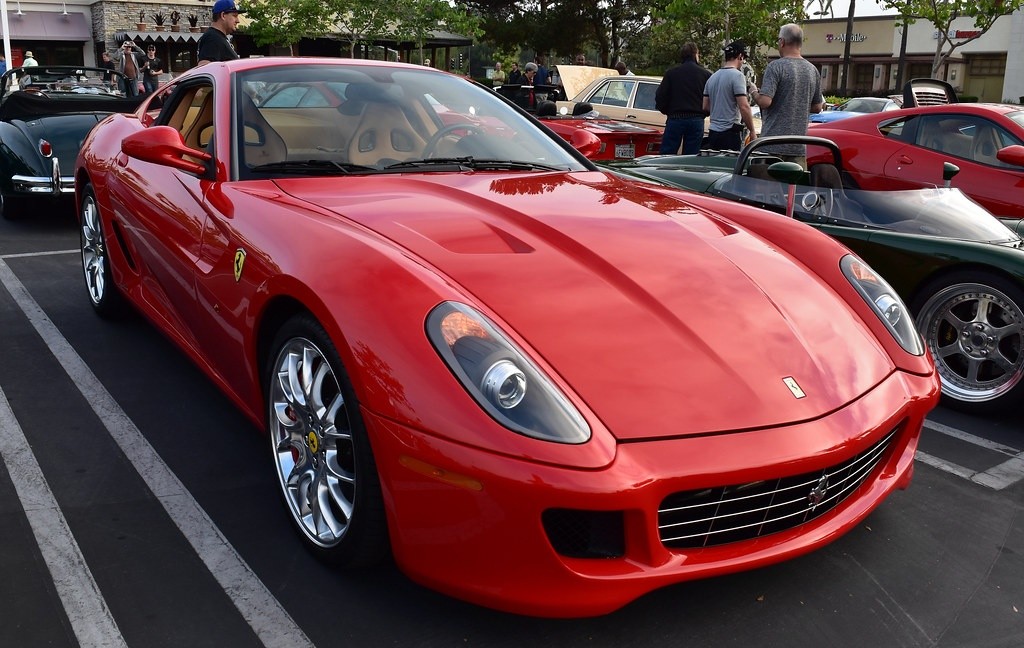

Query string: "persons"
[702, 43, 757, 153]
[197, 0, 256, 99]
[655, 41, 713, 155]
[104, 41, 163, 97]
[748, 23, 822, 173]
[0, 51, 40, 96]
[491, 53, 635, 108]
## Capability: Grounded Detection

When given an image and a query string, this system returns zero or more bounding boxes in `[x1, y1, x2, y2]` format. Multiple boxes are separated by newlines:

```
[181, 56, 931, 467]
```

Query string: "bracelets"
[749, 88, 758, 94]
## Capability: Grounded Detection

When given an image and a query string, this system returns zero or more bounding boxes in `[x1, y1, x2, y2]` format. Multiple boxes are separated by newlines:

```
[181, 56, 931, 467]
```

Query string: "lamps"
[12, 1, 27, 17]
[58, 1, 73, 19]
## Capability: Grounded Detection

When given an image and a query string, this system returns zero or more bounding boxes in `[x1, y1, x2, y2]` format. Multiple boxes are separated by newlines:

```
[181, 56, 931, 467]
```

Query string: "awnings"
[0, 11, 89, 40]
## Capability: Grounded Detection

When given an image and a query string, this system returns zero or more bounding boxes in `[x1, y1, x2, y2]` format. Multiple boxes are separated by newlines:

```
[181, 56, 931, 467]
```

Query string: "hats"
[212, 0, 247, 16]
[147, 45, 156, 50]
[123, 41, 132, 47]
[722, 42, 750, 59]
[24, 51, 34, 57]
[423, 59, 431, 65]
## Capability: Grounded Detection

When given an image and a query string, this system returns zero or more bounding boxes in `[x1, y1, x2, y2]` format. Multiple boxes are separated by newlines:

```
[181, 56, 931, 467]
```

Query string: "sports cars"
[0, 65, 163, 225]
[585, 134, 1024, 415]
[73, 56, 942, 617]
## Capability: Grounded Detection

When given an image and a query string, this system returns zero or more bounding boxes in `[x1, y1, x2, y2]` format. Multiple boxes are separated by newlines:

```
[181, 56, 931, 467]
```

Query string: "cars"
[250, 78, 522, 154]
[809, 96, 904, 125]
[548, 65, 762, 147]
[424, 86, 665, 162]
[743, 100, 1024, 219]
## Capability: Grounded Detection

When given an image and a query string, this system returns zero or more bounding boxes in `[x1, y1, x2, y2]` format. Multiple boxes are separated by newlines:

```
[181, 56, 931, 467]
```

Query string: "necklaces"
[213, 26, 226, 36]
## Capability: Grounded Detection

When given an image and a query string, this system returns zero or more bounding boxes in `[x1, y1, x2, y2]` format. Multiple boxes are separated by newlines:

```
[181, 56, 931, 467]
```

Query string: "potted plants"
[135, 7, 147, 31]
[186, 13, 200, 33]
[168, 10, 181, 32]
[201, 14, 211, 33]
[148, 11, 167, 31]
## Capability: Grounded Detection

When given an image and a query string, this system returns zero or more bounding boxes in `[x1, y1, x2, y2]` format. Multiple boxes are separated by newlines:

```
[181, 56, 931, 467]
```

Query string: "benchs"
[2, 90, 161, 119]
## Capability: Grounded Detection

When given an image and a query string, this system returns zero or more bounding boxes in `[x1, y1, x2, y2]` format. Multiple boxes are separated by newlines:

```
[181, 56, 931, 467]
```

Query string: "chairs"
[343, 101, 429, 165]
[920, 119, 952, 154]
[536, 100, 558, 117]
[970, 123, 1001, 166]
[573, 102, 593, 115]
[746, 163, 804, 212]
[810, 162, 867, 222]
[182, 88, 289, 166]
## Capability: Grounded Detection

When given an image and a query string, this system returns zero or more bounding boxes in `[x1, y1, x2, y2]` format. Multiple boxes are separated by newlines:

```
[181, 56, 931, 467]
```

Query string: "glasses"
[532, 69, 537, 73]
[149, 49, 155, 51]
[775, 38, 782, 45]
